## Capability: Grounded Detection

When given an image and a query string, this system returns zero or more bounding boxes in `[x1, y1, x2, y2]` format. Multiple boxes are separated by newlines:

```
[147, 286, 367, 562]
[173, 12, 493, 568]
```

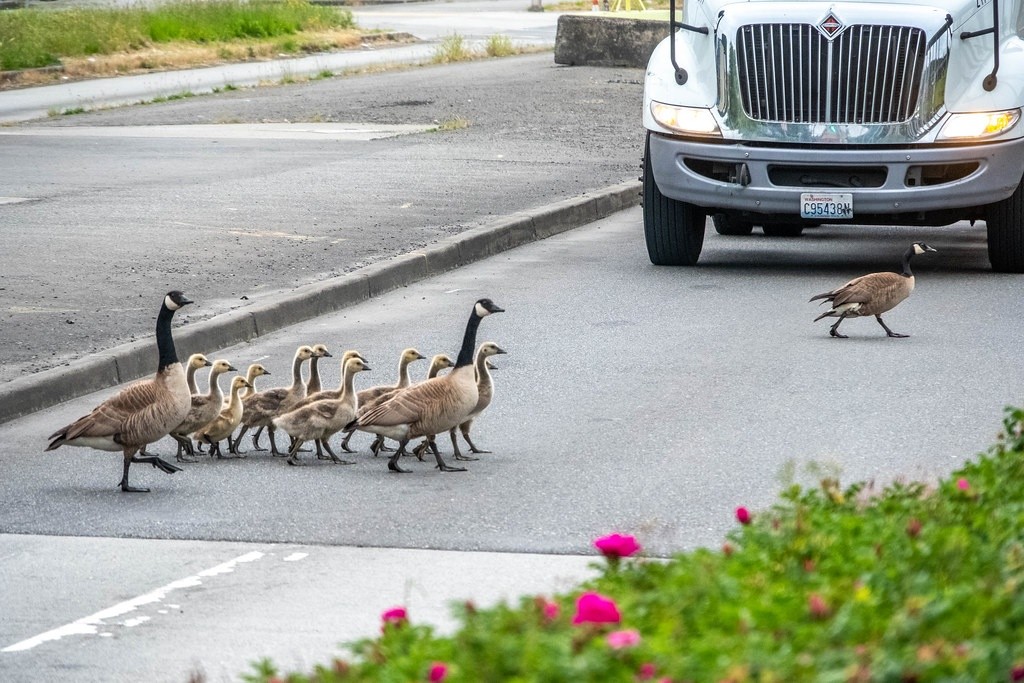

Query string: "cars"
[637, 1, 1024, 276]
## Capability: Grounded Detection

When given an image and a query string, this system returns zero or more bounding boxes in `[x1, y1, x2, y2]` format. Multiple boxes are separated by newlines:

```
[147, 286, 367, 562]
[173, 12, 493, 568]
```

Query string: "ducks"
[45, 291, 510, 494]
[809, 241, 938, 338]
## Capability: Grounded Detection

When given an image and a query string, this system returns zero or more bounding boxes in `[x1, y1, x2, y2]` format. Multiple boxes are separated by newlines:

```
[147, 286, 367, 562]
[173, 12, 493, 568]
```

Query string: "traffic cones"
[592, 0, 601, 12]
